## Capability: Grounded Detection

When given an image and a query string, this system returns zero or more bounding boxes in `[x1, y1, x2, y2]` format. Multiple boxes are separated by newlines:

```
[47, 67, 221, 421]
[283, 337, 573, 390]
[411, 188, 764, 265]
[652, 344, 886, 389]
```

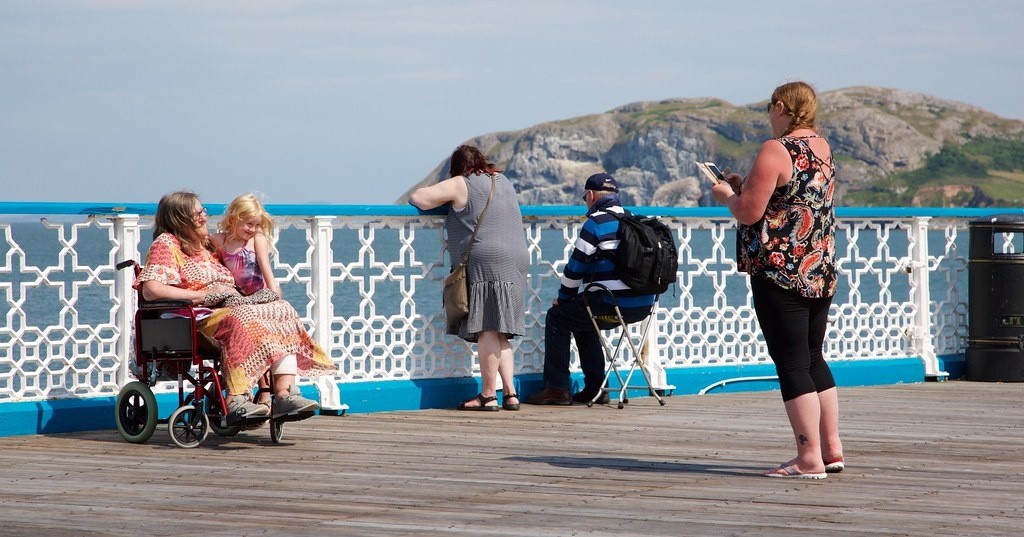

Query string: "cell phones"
[704, 162, 727, 182]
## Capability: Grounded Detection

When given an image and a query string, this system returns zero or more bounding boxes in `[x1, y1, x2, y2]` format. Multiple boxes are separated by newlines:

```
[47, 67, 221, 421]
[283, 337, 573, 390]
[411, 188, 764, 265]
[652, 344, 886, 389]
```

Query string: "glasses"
[766, 102, 773, 113]
[189, 206, 208, 221]
[583, 192, 590, 203]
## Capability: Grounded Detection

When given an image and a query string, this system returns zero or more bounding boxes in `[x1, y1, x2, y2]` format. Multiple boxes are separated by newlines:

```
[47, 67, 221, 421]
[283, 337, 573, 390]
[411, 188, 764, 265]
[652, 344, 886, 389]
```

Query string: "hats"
[584, 173, 619, 193]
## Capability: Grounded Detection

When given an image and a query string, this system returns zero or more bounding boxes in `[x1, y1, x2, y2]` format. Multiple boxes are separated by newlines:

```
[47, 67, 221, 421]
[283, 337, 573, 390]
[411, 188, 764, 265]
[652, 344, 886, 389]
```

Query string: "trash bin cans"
[964, 214, 1024, 382]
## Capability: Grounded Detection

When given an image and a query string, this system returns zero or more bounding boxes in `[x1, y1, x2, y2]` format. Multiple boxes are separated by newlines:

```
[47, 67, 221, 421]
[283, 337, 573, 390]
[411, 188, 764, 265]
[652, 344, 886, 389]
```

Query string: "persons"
[132, 192, 320, 428]
[711, 81, 845, 480]
[526, 172, 655, 406]
[409, 145, 531, 411]
[210, 193, 281, 415]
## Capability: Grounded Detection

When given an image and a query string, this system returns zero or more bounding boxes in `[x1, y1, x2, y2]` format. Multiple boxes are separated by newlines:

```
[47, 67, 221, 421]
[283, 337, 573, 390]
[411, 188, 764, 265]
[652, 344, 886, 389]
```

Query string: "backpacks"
[596, 205, 678, 294]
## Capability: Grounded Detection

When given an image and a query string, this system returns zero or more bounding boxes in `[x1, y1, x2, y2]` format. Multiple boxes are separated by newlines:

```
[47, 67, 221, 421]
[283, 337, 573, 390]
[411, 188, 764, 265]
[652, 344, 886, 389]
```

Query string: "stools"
[584, 283, 666, 409]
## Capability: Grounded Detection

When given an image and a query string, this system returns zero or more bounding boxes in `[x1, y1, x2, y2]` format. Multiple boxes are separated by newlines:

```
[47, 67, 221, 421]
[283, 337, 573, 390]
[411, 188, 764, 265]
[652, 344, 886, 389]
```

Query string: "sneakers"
[220, 394, 268, 428]
[272, 384, 320, 420]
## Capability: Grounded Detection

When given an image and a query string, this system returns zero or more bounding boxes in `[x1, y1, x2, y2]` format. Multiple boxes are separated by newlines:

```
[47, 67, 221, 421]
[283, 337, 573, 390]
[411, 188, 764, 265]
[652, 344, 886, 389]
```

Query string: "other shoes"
[525, 387, 573, 405]
[571, 387, 611, 404]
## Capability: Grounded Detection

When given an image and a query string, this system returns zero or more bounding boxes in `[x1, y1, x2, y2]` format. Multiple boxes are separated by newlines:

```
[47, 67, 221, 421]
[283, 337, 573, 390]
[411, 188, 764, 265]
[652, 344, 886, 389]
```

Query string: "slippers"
[764, 461, 845, 480]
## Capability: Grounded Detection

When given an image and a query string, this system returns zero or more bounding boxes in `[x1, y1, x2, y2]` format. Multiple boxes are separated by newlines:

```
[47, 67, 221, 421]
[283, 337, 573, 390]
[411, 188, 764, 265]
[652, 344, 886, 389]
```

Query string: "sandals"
[501, 393, 521, 410]
[457, 392, 499, 411]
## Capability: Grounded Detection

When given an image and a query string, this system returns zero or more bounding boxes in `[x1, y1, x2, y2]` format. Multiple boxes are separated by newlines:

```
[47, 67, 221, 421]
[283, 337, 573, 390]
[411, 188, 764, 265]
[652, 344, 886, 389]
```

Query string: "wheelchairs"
[113, 259, 315, 450]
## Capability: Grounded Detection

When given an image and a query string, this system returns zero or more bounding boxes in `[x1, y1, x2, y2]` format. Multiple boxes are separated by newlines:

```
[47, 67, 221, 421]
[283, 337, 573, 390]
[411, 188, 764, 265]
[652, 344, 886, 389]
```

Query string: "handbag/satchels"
[442, 263, 470, 323]
